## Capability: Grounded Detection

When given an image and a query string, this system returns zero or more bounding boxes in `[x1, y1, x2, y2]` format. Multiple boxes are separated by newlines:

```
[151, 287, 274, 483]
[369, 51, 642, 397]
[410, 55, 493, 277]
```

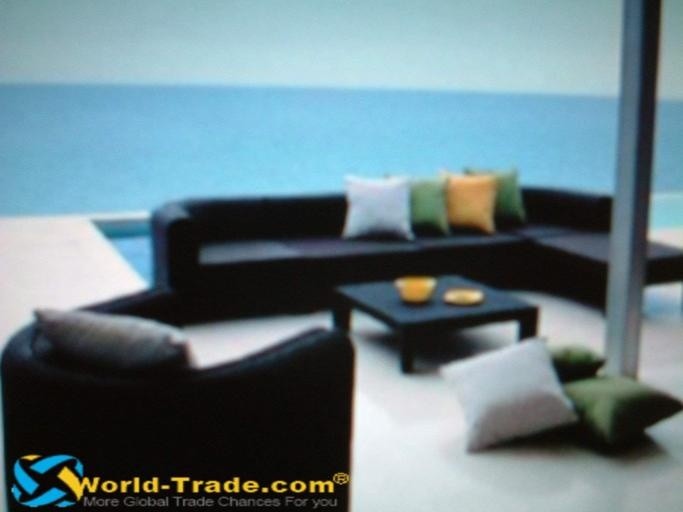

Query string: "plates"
[443, 288, 486, 307]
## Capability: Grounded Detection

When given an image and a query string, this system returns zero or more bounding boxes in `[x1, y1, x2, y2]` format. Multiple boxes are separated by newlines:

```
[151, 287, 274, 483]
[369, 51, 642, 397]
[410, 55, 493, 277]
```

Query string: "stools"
[534, 235, 682, 316]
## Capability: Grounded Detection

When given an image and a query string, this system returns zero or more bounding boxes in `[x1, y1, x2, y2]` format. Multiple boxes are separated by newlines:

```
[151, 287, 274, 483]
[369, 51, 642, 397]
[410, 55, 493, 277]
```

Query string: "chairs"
[0, 285, 355, 512]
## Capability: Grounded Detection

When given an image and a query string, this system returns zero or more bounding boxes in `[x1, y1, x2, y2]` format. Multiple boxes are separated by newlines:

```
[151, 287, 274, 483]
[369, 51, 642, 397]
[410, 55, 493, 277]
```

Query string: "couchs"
[152, 189, 611, 320]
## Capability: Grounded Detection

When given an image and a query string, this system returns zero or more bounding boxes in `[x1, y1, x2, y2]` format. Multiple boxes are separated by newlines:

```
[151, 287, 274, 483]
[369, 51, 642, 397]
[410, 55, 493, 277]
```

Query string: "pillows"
[30, 309, 188, 370]
[439, 336, 683, 452]
[341, 169, 526, 242]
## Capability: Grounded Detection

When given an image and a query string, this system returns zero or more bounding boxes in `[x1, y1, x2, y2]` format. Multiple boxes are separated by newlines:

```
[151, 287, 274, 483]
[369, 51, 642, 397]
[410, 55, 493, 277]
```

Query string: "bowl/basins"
[395, 273, 437, 306]
